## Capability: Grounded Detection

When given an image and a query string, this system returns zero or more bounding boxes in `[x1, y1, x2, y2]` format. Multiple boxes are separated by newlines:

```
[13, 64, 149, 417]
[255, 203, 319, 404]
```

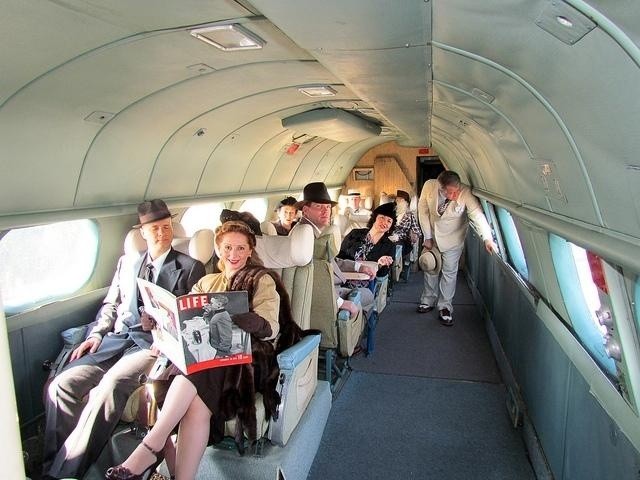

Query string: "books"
[136, 278, 252, 376]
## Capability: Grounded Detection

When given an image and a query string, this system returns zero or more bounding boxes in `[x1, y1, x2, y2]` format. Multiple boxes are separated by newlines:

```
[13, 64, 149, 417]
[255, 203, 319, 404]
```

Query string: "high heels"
[105, 442, 167, 479]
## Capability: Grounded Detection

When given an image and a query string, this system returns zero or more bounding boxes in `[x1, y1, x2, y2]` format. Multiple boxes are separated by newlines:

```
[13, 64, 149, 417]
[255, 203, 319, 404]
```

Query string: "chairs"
[46, 228, 214, 430]
[336, 214, 389, 314]
[338, 194, 373, 211]
[138, 225, 320, 445]
[381, 193, 421, 282]
[260, 220, 376, 357]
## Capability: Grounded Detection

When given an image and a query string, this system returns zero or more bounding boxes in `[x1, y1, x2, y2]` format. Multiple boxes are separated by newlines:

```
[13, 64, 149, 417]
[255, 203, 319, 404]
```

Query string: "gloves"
[230, 312, 272, 339]
[141, 312, 155, 331]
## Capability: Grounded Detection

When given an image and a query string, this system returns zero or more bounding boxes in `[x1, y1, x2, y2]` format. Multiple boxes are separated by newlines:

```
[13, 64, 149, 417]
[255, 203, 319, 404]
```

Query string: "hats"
[292, 182, 338, 211]
[133, 199, 180, 230]
[341, 188, 366, 198]
[419, 245, 441, 278]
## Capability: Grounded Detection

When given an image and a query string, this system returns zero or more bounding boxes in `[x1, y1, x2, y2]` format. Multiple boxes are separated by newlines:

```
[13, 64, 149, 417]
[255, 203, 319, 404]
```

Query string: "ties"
[142, 263, 154, 283]
[437, 199, 451, 215]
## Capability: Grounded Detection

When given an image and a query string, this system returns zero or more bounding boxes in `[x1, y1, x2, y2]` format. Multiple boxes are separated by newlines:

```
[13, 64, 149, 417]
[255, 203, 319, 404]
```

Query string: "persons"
[343, 188, 372, 228]
[105, 209, 280, 480]
[337, 203, 397, 293]
[417, 170, 499, 326]
[43, 199, 206, 480]
[204, 295, 232, 359]
[271, 197, 299, 238]
[288, 182, 374, 320]
[394, 189, 423, 256]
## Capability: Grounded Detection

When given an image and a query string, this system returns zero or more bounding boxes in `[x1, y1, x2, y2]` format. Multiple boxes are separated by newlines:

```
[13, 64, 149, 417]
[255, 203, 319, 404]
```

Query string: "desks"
[339, 260, 378, 281]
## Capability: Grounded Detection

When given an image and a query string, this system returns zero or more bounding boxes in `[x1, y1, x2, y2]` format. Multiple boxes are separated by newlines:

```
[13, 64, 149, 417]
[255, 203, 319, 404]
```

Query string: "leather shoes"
[439, 309, 454, 326]
[416, 304, 435, 313]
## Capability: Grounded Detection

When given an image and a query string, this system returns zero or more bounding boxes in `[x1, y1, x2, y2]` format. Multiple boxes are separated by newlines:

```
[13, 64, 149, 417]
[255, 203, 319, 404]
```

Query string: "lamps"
[380, 126, 389, 133]
[299, 85, 337, 98]
[189, 24, 266, 53]
[358, 108, 377, 114]
